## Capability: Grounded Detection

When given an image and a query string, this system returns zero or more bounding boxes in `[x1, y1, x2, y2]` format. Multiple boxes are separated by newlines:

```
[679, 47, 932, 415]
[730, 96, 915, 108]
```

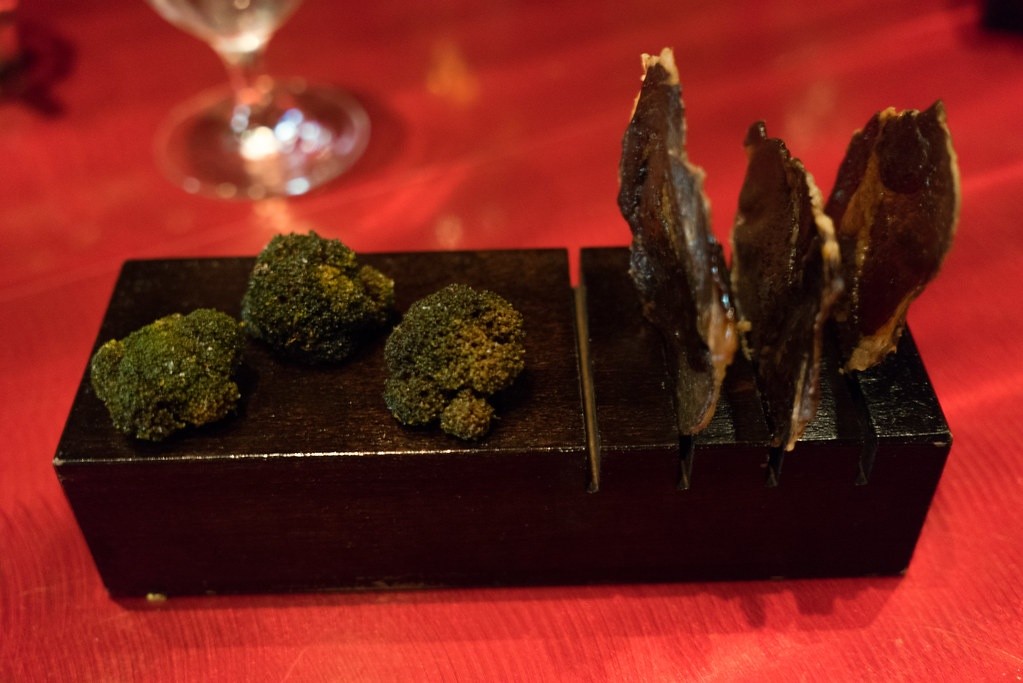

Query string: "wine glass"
[150, 0, 373, 201]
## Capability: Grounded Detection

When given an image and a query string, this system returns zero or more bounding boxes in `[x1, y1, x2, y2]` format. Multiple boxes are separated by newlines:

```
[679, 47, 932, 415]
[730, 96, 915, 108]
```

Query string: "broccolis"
[383, 284, 527, 441]
[92, 308, 246, 439]
[241, 232, 394, 361]
